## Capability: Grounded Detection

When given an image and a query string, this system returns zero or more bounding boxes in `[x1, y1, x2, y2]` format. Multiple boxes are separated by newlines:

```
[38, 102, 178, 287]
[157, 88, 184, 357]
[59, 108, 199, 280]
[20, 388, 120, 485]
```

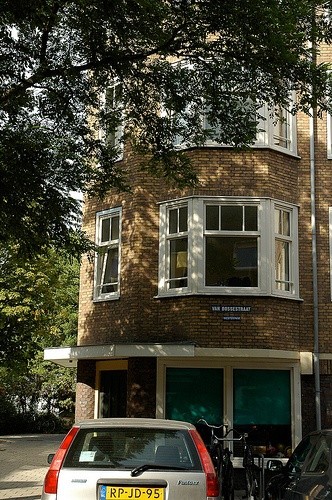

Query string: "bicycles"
[197, 418, 259, 500]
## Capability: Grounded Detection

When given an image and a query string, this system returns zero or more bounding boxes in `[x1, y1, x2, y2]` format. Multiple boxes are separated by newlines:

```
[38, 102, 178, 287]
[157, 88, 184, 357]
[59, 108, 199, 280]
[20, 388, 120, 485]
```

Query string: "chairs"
[156, 446, 179, 463]
[88, 435, 114, 457]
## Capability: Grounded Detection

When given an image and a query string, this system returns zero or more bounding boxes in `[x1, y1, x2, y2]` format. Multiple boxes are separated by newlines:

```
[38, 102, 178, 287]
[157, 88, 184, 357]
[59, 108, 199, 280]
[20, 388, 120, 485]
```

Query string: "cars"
[264, 429, 332, 500]
[41, 417, 221, 500]
